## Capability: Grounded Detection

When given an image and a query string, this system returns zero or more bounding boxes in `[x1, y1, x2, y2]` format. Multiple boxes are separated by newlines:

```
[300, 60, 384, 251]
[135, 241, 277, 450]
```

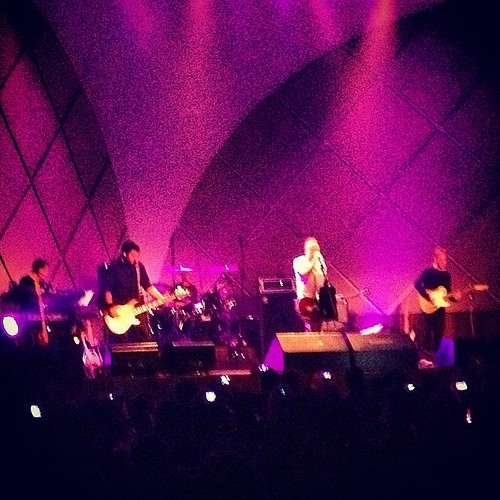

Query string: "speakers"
[259, 293, 418, 374]
[434, 334, 500, 368]
[110, 341, 216, 373]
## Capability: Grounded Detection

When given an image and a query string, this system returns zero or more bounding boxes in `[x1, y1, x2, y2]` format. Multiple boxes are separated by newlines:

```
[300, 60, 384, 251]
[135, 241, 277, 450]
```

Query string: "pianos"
[2, 287, 86, 333]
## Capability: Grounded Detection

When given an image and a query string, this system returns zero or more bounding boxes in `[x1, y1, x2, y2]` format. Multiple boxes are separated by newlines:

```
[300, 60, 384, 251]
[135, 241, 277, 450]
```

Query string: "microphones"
[319, 258, 324, 269]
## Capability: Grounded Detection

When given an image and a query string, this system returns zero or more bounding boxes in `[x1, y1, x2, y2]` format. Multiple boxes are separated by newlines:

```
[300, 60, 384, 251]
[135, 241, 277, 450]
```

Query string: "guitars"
[298, 286, 372, 320]
[80, 319, 104, 369]
[418, 284, 489, 314]
[103, 286, 191, 335]
[26, 268, 49, 346]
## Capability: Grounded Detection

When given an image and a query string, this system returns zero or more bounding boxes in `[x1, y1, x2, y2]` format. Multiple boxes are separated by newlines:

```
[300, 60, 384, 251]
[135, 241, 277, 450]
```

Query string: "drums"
[181, 315, 223, 344]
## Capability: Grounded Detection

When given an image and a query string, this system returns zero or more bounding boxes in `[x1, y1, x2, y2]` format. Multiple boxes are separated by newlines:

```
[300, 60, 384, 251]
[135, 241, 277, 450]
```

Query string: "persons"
[414, 247, 462, 358]
[103, 240, 168, 344]
[0, 366, 500, 500]
[293, 238, 327, 333]
[19, 257, 50, 344]
